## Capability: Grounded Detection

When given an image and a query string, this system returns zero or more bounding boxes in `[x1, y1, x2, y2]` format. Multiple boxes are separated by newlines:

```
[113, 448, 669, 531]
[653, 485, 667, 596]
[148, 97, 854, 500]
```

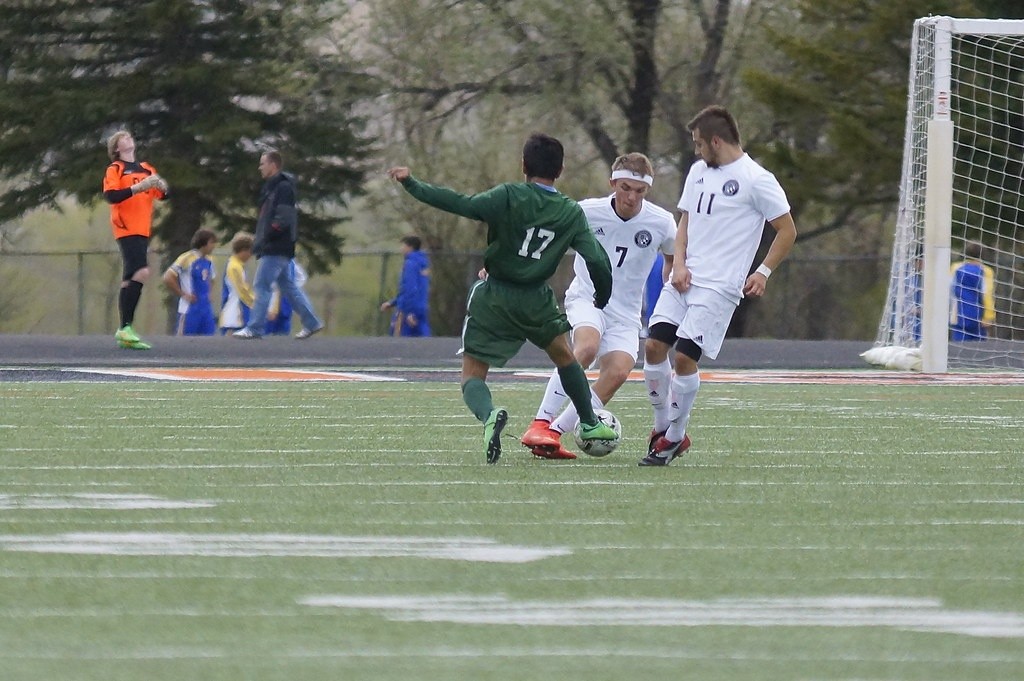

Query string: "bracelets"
[754, 264, 772, 279]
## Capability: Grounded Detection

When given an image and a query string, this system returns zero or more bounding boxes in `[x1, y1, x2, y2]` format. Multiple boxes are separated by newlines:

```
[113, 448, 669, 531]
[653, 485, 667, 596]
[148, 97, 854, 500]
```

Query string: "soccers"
[573, 408, 622, 458]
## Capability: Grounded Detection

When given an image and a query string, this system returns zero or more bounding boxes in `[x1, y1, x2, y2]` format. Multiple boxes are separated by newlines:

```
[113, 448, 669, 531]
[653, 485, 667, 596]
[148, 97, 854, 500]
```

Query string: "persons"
[637, 103, 797, 468]
[381, 236, 430, 338]
[103, 131, 168, 349]
[161, 230, 306, 338]
[521, 152, 678, 459]
[950, 242, 995, 341]
[388, 131, 619, 462]
[232, 149, 325, 339]
[887, 242, 923, 347]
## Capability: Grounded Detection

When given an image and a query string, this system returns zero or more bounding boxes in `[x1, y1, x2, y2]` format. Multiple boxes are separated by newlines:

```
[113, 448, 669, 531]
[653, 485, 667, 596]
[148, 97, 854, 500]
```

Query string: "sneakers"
[638, 429, 692, 467]
[233, 328, 262, 339]
[520, 420, 577, 460]
[114, 327, 148, 349]
[578, 420, 620, 440]
[294, 322, 321, 340]
[482, 407, 508, 464]
